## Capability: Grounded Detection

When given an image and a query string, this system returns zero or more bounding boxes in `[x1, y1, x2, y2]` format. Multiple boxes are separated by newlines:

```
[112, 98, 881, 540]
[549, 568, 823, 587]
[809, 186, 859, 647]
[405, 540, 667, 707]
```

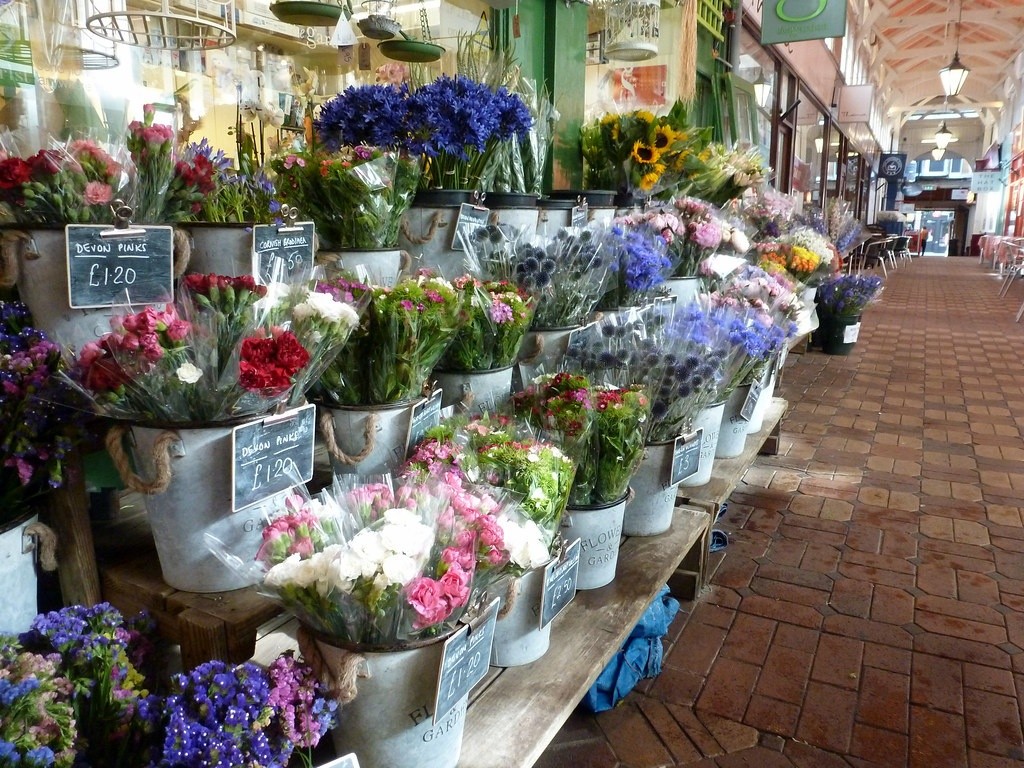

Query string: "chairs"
[845, 231, 913, 276]
[978, 236, 1024, 323]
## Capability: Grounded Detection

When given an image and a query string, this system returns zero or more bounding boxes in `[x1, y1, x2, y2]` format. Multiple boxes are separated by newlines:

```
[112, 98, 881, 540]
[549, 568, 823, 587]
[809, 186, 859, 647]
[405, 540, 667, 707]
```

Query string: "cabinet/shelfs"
[41, 460, 710, 767]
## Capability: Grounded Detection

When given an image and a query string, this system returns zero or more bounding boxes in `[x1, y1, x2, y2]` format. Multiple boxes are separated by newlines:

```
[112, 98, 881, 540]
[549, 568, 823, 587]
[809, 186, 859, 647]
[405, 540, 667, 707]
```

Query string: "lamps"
[939, 0, 970, 96]
[931, 146, 946, 162]
[934, 97, 953, 150]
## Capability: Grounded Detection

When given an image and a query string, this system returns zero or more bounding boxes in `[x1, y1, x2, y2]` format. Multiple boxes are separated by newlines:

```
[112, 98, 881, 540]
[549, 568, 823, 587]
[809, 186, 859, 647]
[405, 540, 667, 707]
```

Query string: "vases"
[0, 191, 865, 768]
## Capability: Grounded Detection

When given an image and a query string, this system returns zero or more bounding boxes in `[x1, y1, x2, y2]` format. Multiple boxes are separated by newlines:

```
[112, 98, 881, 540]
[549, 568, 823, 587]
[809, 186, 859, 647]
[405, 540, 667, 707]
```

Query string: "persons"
[918, 226, 928, 257]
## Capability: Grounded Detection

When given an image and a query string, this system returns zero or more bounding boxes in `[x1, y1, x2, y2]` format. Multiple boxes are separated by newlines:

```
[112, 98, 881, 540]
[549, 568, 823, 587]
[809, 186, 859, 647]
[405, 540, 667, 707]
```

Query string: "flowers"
[248, 464, 511, 645]
[0, 586, 149, 768]
[136, 648, 342, 768]
[0, 74, 883, 489]
